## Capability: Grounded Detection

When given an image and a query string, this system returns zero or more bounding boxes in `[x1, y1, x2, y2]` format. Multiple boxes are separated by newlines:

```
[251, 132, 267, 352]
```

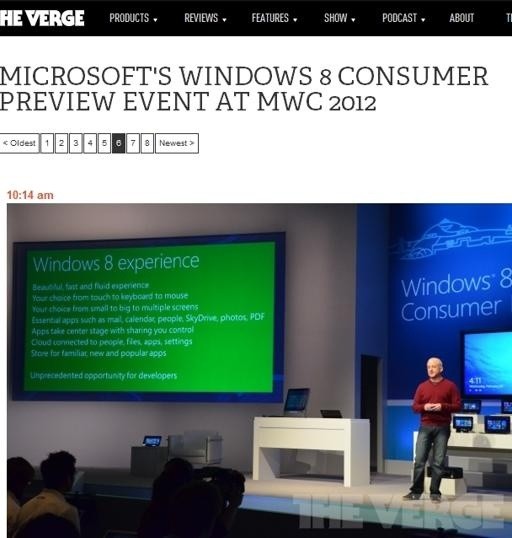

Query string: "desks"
[256, 417, 370, 489]
[413, 415, 512, 500]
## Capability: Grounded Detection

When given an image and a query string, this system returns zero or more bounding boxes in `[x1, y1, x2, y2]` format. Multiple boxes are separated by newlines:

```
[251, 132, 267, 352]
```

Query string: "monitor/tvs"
[453, 417, 473, 431]
[320, 409, 343, 418]
[502, 400, 512, 414]
[283, 387, 311, 411]
[143, 435, 162, 447]
[461, 399, 481, 414]
[484, 415, 510, 433]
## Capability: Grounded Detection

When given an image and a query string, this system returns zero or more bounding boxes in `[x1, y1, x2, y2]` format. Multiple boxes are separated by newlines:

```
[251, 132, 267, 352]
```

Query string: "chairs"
[170, 432, 221, 479]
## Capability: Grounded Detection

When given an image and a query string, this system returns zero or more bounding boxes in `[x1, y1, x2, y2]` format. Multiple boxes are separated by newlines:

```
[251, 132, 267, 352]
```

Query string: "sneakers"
[401, 491, 422, 501]
[429, 492, 442, 505]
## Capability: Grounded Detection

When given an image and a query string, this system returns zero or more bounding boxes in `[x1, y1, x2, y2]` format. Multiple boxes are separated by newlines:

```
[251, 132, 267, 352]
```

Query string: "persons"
[8, 450, 246, 537]
[402, 356, 461, 501]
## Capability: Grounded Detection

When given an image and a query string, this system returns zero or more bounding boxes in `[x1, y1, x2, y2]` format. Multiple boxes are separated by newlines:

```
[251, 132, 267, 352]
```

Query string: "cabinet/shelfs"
[131, 447, 169, 483]
